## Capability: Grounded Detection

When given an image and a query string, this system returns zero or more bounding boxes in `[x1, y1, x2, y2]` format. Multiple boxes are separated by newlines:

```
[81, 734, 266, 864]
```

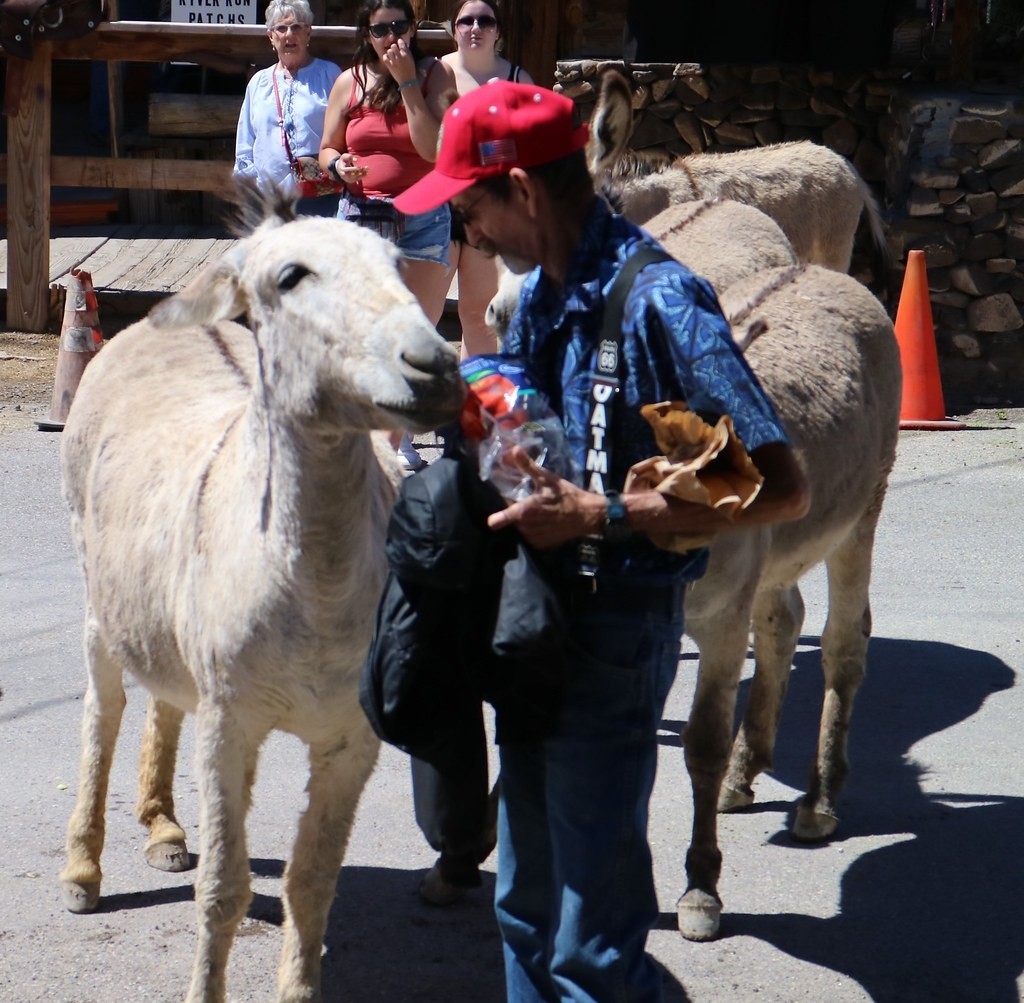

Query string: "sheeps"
[481, 71, 892, 346]
[667, 263, 903, 939]
[628, 196, 803, 303]
[61, 169, 471, 1003]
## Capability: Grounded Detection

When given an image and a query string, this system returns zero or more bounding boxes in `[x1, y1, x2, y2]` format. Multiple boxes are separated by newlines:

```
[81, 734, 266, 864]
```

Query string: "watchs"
[604, 490, 633, 540]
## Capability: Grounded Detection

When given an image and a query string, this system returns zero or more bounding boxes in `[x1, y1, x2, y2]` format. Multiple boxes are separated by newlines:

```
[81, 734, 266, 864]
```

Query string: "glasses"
[455, 16, 497, 33]
[272, 22, 308, 36]
[368, 20, 410, 38]
[452, 179, 505, 226]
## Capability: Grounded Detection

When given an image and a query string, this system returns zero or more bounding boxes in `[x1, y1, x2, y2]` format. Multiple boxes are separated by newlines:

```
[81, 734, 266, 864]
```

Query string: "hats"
[392, 81, 589, 215]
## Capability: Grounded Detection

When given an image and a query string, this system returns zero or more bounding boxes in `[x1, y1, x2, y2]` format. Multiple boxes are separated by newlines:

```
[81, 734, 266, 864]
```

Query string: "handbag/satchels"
[290, 154, 345, 198]
[349, 452, 505, 768]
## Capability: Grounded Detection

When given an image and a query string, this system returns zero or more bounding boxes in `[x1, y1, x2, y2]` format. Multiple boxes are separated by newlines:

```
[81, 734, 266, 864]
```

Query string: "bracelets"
[327, 156, 344, 183]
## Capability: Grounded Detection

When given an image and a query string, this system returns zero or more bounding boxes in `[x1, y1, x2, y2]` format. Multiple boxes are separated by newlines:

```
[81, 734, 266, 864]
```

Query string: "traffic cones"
[33, 266, 104, 429]
[892, 249, 969, 431]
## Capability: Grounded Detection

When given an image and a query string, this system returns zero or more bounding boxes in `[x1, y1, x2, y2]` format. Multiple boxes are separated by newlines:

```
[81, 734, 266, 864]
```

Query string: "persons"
[233, 0, 343, 217]
[319, 0, 460, 469]
[422, 0, 534, 362]
[392, 77, 811, 1003]
